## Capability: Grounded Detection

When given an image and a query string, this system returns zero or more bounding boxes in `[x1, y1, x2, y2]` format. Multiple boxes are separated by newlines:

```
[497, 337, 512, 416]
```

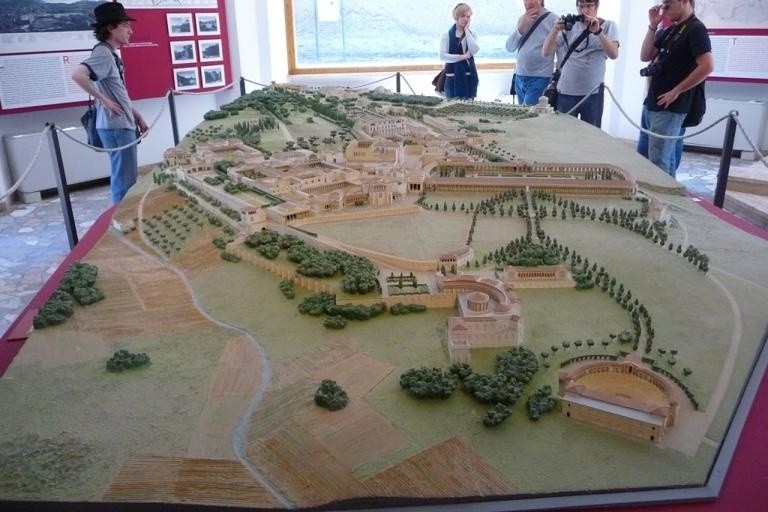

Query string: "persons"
[505, 0, 561, 106]
[70, 2, 149, 207]
[637, 0, 714, 181]
[438, 2, 481, 101]
[542, 0, 620, 129]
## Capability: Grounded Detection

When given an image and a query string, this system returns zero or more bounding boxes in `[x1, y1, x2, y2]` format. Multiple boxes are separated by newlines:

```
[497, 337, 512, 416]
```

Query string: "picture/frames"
[166, 12, 226, 91]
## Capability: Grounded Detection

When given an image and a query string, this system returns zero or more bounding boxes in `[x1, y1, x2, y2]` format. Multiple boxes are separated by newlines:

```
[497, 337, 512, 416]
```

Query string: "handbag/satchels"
[80, 106, 104, 152]
[432, 69, 447, 93]
[543, 84, 559, 111]
[510, 74, 516, 96]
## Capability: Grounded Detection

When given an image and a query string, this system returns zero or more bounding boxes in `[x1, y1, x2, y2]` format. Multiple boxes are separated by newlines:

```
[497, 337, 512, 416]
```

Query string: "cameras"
[640, 57, 663, 77]
[561, 13, 584, 31]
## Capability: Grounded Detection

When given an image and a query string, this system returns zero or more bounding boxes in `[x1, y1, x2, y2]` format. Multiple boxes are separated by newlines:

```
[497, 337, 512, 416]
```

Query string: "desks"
[682, 98, 767, 160]
[3, 126, 110, 203]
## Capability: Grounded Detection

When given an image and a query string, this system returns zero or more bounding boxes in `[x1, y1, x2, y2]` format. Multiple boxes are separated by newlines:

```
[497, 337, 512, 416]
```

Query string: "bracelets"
[647, 24, 658, 32]
[593, 28, 603, 36]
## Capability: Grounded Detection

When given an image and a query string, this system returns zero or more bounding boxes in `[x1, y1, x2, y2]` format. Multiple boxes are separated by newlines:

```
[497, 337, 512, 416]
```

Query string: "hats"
[89, 2, 137, 27]
